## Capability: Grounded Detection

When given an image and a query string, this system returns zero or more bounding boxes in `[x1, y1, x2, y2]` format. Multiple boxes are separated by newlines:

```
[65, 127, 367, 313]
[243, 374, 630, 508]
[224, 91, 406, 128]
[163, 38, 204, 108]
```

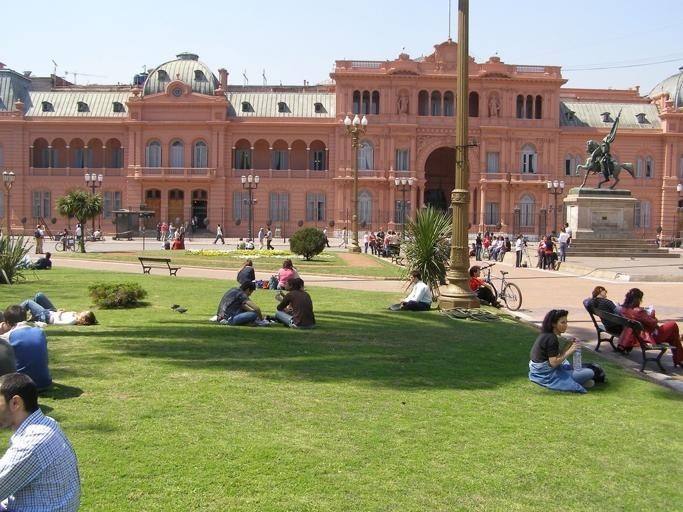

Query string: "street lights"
[241, 175, 259, 241]
[85, 173, 103, 232]
[547, 180, 564, 236]
[395, 178, 414, 239]
[344, 114, 368, 253]
[3, 170, 16, 240]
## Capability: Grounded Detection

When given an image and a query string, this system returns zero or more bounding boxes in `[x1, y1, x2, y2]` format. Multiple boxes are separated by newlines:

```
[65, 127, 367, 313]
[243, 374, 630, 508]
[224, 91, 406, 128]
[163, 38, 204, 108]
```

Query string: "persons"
[34, 225, 45, 254]
[0, 310, 16, 376]
[400, 270, 432, 311]
[63, 229, 68, 236]
[156, 221, 185, 241]
[0, 305, 52, 392]
[322, 226, 330, 248]
[216, 280, 270, 326]
[19, 292, 96, 326]
[587, 286, 623, 336]
[276, 259, 299, 289]
[191, 216, 198, 230]
[237, 238, 254, 250]
[264, 228, 274, 250]
[528, 309, 594, 393]
[93, 230, 101, 240]
[470, 223, 572, 271]
[76, 225, 81, 240]
[338, 226, 347, 249]
[257, 228, 264, 250]
[275, 278, 316, 329]
[469, 266, 504, 309]
[25, 252, 52, 271]
[364, 226, 401, 259]
[213, 224, 225, 244]
[0, 372, 81, 512]
[236, 259, 255, 284]
[619, 288, 683, 369]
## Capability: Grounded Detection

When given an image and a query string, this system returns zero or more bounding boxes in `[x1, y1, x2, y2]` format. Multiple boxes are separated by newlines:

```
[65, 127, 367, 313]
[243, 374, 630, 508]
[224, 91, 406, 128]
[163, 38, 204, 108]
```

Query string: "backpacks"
[34, 229, 41, 238]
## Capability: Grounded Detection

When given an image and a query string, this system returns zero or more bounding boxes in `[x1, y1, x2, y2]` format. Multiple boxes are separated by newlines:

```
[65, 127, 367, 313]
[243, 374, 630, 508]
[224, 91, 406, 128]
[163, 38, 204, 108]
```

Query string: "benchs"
[391, 253, 404, 265]
[138, 256, 181, 277]
[583, 300, 679, 373]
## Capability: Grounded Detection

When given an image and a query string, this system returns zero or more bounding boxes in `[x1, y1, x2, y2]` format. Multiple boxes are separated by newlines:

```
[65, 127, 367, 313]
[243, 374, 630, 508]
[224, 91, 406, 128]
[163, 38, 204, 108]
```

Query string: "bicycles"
[478, 262, 522, 311]
[54, 235, 79, 252]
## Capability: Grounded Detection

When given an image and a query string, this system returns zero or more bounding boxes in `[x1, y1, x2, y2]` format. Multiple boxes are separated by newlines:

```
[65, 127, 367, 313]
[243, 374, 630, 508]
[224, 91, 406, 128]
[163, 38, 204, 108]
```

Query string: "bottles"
[573, 339, 582, 373]
